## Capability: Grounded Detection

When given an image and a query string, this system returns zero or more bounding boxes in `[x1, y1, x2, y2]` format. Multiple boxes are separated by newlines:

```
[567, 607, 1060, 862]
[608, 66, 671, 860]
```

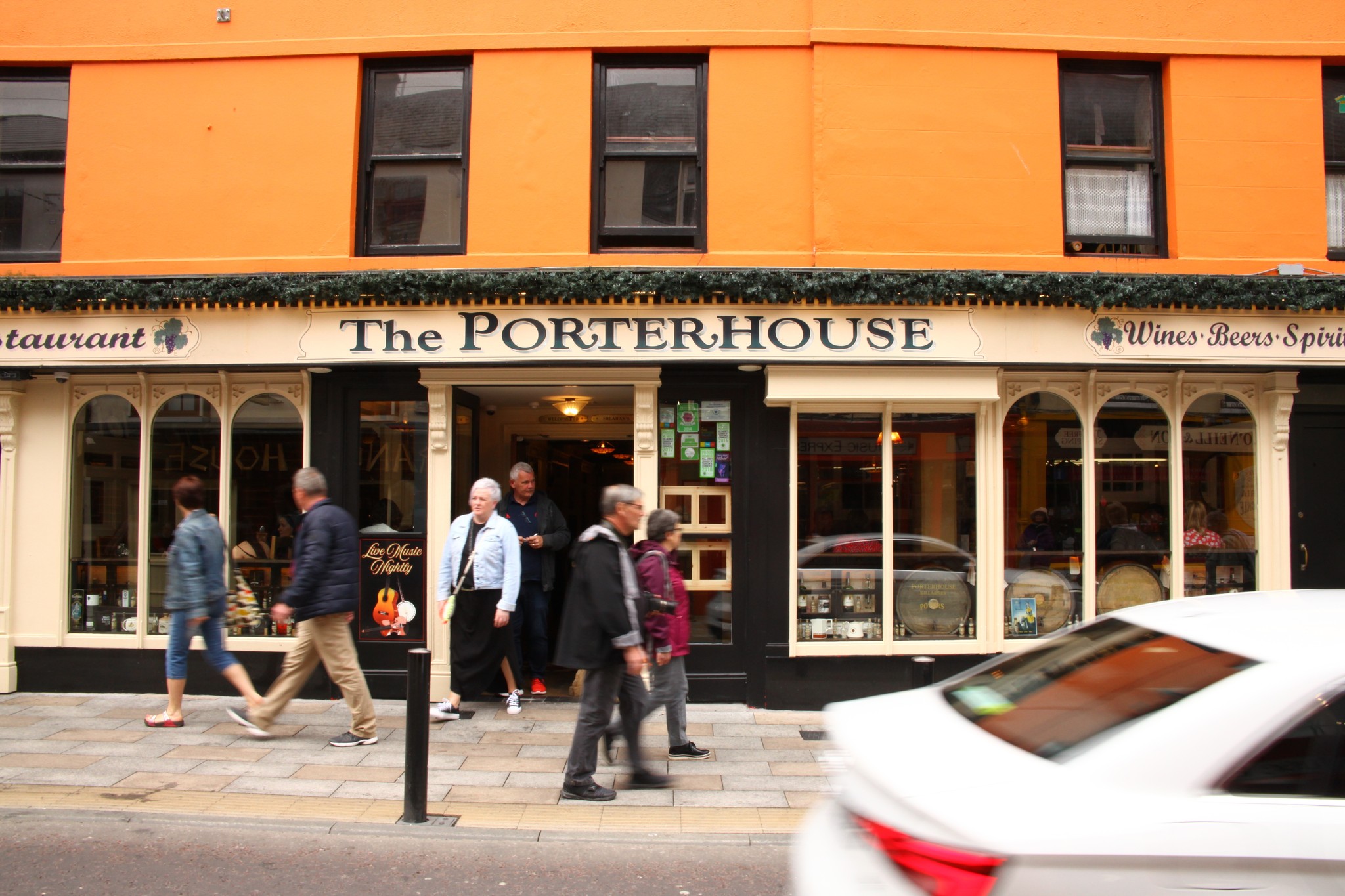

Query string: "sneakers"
[494, 689, 524, 697]
[531, 675, 547, 695]
[506, 689, 522, 714]
[225, 706, 271, 737]
[429, 698, 460, 720]
[328, 731, 378, 747]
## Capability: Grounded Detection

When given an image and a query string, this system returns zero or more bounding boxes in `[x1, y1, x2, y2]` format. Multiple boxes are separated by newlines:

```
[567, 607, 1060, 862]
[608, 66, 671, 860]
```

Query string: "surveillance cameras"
[485, 404, 497, 415]
[53, 371, 70, 384]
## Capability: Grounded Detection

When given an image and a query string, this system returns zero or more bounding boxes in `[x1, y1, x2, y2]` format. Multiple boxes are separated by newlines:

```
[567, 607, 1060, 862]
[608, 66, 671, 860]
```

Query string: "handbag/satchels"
[440, 595, 456, 624]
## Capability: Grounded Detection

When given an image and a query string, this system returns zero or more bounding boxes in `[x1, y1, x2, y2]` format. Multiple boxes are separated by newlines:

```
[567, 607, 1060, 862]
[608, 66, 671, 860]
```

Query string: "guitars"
[361, 616, 410, 637]
[397, 571, 416, 623]
[372, 565, 399, 625]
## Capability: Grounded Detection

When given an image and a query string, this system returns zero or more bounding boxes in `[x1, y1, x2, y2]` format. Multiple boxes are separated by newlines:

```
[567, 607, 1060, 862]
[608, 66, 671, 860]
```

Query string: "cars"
[782, 588, 1345, 896]
[702, 531, 1019, 637]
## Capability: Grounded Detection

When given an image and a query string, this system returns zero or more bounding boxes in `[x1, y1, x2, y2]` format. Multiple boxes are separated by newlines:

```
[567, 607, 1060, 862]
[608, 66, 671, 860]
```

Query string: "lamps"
[553, 398, 590, 419]
[306, 366, 331, 374]
[876, 432, 903, 446]
[590, 439, 634, 466]
[739, 364, 763, 372]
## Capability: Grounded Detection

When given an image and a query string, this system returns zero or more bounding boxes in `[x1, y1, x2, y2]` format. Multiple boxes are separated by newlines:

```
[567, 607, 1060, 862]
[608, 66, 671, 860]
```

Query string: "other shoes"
[627, 768, 671, 789]
[560, 782, 617, 801]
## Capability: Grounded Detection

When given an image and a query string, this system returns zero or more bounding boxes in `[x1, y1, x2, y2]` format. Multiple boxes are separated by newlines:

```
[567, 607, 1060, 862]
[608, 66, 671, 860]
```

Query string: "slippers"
[144, 710, 184, 728]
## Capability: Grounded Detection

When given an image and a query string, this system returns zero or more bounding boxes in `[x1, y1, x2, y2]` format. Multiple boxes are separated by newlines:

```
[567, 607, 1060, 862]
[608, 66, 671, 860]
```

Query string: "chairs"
[1220, 529, 1252, 550]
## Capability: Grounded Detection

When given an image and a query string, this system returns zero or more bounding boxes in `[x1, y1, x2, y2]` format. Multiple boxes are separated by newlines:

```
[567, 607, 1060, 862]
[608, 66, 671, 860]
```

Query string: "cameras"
[634, 585, 678, 616]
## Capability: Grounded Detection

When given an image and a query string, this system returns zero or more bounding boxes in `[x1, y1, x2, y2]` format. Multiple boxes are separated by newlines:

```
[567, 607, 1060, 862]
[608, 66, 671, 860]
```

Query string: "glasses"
[624, 502, 642, 509]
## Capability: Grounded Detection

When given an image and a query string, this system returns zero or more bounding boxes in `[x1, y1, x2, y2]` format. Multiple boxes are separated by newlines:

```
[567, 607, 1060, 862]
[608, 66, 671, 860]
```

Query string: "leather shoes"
[668, 740, 711, 760]
[602, 725, 615, 763]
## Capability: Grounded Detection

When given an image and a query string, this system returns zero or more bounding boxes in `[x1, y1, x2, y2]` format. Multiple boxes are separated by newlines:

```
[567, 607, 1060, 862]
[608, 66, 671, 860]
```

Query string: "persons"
[1016, 506, 1059, 551]
[492, 460, 571, 697]
[223, 468, 379, 747]
[256, 513, 299, 560]
[1181, 499, 1226, 550]
[142, 475, 266, 729]
[1206, 511, 1251, 549]
[1096, 501, 1157, 551]
[552, 482, 685, 804]
[429, 477, 524, 720]
[601, 509, 711, 765]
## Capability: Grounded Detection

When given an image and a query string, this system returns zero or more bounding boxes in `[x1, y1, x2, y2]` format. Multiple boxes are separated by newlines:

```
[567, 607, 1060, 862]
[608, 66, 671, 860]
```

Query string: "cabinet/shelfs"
[796, 564, 883, 638]
[1183, 558, 1251, 598]
